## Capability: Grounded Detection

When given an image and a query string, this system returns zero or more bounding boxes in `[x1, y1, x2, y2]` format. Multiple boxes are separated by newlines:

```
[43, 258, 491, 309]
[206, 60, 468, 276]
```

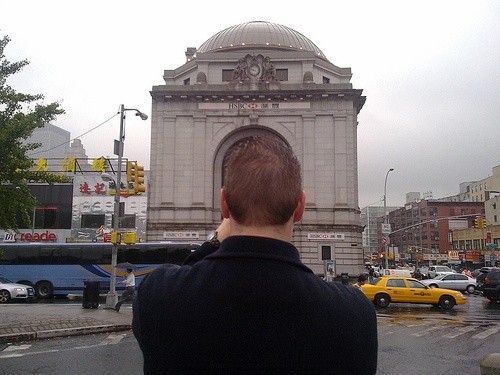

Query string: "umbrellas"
[113, 262, 138, 275]
[364, 264, 372, 266]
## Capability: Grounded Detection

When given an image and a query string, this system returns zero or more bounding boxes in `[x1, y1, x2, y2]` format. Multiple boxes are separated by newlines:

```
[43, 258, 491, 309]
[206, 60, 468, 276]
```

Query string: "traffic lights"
[480, 218, 487, 229]
[134, 165, 145, 194]
[126, 161, 135, 183]
[474, 218, 479, 229]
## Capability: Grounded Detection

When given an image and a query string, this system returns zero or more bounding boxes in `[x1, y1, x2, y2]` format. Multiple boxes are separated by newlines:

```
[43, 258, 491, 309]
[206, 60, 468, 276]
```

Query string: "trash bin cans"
[81, 279, 102, 311]
[340, 273, 351, 286]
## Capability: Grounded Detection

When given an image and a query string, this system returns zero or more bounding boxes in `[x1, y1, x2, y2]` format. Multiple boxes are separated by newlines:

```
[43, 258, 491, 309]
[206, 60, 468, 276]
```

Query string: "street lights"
[383, 168, 395, 270]
[104, 104, 149, 308]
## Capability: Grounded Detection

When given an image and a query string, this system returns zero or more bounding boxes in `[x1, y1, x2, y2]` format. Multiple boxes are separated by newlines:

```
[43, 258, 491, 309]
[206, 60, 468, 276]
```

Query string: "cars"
[359, 275, 468, 310]
[364, 261, 481, 280]
[0, 276, 36, 304]
[419, 272, 477, 294]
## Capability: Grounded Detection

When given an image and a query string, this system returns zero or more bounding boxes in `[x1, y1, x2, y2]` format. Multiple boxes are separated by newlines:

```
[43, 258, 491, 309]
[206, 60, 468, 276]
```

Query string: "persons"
[351, 274, 367, 293]
[368, 267, 374, 280]
[132, 136, 378, 375]
[462, 268, 471, 276]
[112, 269, 135, 312]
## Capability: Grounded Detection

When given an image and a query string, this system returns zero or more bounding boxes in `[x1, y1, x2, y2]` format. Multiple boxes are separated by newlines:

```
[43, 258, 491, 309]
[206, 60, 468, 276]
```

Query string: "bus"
[0, 241, 204, 300]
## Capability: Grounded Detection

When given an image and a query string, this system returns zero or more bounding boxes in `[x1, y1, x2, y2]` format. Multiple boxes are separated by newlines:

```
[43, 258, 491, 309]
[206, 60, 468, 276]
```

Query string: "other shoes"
[112, 307, 120, 312]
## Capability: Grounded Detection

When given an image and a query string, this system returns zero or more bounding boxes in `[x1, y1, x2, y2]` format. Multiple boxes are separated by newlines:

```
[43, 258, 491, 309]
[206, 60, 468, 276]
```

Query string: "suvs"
[475, 267, 500, 304]
